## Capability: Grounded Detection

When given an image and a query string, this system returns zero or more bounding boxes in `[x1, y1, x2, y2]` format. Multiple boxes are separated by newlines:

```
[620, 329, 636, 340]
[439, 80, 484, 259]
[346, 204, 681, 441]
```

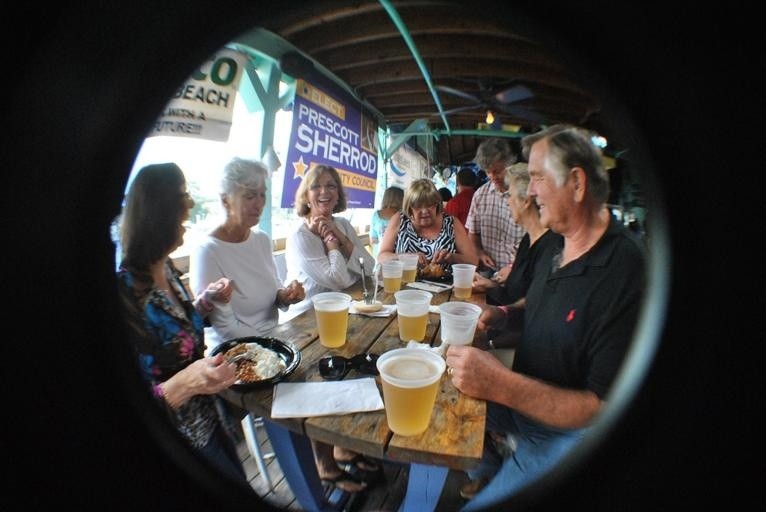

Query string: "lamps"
[262, 144, 281, 180]
[486, 110, 496, 125]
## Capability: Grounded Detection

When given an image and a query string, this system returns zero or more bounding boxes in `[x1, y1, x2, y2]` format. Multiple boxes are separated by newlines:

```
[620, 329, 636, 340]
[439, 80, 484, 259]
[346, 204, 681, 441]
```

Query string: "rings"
[222, 381, 228, 390]
[447, 367, 454, 375]
[326, 223, 329, 228]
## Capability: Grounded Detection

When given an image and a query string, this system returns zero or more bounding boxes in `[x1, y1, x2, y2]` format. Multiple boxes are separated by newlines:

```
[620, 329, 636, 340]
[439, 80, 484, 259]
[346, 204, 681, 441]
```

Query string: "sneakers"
[459, 479, 488, 499]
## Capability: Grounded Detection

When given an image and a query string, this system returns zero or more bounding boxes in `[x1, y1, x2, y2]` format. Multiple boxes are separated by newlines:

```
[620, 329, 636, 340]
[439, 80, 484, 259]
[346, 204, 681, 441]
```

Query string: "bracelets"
[340, 236, 350, 248]
[197, 293, 213, 312]
[322, 235, 339, 244]
[498, 305, 510, 328]
[160, 383, 179, 410]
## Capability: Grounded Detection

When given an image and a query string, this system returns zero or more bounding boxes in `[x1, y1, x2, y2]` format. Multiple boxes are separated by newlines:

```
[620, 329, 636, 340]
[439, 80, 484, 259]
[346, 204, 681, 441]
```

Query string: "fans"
[431, 69, 545, 125]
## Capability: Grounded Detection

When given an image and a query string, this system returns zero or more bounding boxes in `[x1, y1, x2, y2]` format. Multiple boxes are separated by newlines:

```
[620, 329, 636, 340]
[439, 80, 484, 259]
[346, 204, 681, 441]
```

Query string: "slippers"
[332, 453, 383, 473]
[319, 470, 368, 493]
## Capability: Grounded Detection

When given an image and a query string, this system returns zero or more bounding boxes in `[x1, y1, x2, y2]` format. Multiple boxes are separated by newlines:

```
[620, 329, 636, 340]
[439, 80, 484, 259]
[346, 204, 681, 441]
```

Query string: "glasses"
[179, 188, 190, 198]
[309, 183, 337, 191]
[318, 353, 381, 381]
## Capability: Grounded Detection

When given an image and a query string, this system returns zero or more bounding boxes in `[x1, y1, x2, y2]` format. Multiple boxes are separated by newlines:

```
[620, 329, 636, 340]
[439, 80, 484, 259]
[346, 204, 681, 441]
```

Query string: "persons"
[366, 135, 531, 285]
[438, 122, 650, 511]
[188, 158, 382, 496]
[119, 161, 250, 484]
[281, 161, 380, 319]
[447, 162, 562, 351]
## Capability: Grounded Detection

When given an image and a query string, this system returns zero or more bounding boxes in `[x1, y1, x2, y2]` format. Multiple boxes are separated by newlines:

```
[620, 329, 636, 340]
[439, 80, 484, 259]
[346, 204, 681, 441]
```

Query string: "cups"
[311, 291, 352, 349]
[451, 264, 477, 299]
[394, 290, 433, 342]
[381, 260, 403, 293]
[398, 254, 419, 282]
[439, 302, 483, 358]
[376, 347, 447, 437]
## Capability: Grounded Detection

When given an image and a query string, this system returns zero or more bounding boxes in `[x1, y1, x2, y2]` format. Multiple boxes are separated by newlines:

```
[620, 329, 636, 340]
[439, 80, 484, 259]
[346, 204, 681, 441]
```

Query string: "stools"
[238, 409, 280, 496]
[487, 347, 515, 369]
[502, 432, 517, 462]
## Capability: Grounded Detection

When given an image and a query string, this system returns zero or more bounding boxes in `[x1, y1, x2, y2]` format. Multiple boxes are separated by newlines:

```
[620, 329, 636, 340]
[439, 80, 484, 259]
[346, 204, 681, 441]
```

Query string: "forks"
[359, 257, 372, 304]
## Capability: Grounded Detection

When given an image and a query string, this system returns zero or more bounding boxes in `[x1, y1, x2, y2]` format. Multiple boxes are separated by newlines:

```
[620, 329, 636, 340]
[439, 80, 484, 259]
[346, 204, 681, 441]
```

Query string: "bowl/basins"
[208, 335, 301, 392]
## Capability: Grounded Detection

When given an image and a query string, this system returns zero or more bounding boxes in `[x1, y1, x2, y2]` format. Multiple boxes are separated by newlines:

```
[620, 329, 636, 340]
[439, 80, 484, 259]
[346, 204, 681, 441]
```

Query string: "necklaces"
[154, 284, 171, 294]
